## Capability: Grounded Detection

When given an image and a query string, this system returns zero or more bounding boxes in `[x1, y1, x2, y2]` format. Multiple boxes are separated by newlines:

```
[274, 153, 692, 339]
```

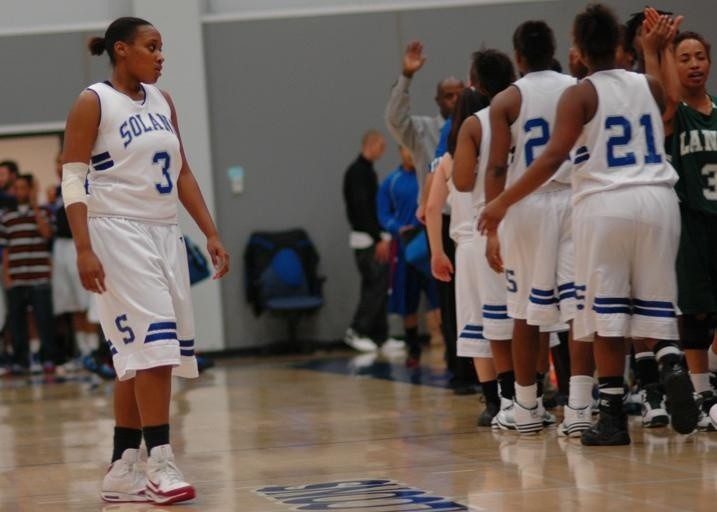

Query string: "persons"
[1, 150, 117, 386]
[335, 1, 716, 446]
[58, 17, 232, 505]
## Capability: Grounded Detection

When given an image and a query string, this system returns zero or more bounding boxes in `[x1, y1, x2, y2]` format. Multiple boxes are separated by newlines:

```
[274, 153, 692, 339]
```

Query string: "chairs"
[242, 228, 326, 353]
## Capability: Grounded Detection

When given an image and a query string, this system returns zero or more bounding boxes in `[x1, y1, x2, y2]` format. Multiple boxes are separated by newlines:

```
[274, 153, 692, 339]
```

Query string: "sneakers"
[342, 327, 444, 368]
[477, 356, 717, 446]
[0, 351, 117, 380]
[101, 448, 147, 502]
[146, 444, 196, 505]
